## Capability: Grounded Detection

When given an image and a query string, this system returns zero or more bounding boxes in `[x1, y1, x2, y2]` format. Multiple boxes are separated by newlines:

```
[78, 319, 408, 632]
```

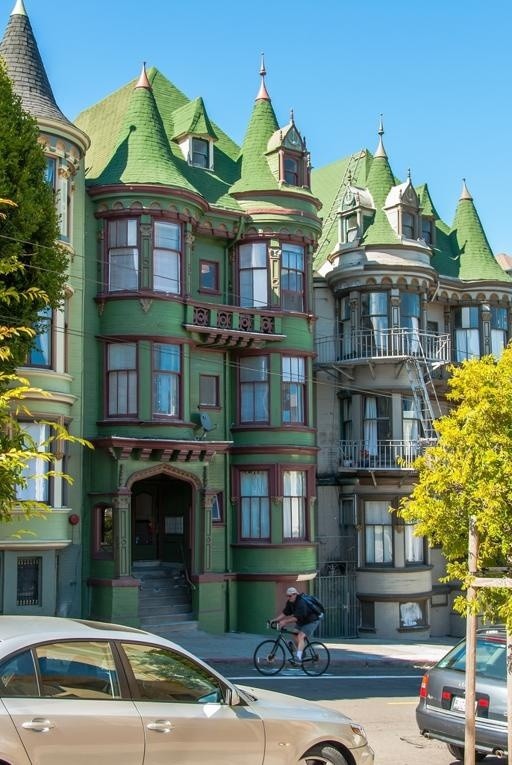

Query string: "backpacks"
[299, 593, 325, 617]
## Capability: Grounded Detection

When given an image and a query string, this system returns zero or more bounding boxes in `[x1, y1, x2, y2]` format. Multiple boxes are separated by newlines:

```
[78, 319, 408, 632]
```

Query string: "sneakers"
[289, 657, 303, 664]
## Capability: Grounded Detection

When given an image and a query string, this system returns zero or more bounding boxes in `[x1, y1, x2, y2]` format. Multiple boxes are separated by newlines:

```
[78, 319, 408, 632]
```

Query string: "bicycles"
[252, 619, 331, 677]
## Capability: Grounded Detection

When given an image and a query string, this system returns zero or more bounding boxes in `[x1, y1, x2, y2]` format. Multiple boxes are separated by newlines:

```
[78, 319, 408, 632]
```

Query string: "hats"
[287, 587, 300, 596]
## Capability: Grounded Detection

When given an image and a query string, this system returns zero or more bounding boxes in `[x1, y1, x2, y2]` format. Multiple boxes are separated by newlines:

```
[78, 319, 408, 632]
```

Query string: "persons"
[266, 586, 326, 667]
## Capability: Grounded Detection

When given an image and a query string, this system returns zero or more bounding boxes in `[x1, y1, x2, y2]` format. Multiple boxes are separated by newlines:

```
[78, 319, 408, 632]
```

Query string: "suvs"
[415, 623, 509, 765]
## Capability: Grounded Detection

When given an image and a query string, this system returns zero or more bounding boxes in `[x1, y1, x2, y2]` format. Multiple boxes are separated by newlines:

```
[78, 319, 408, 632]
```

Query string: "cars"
[0, 613, 376, 765]
